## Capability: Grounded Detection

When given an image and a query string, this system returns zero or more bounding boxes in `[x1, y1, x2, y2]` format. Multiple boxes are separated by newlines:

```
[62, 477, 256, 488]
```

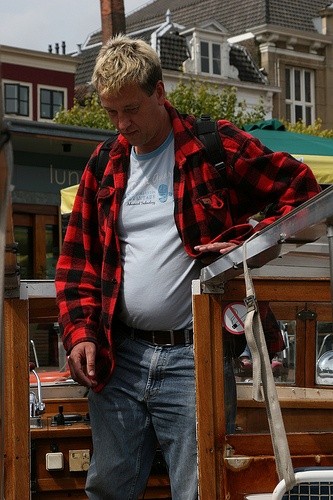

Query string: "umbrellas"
[59, 118, 333, 215]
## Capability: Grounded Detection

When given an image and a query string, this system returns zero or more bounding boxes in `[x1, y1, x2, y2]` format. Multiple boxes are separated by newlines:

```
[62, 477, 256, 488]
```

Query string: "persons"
[55, 33, 323, 500]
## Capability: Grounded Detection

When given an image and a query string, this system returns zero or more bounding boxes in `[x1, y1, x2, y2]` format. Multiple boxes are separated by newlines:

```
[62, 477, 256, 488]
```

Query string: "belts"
[117, 322, 193, 347]
